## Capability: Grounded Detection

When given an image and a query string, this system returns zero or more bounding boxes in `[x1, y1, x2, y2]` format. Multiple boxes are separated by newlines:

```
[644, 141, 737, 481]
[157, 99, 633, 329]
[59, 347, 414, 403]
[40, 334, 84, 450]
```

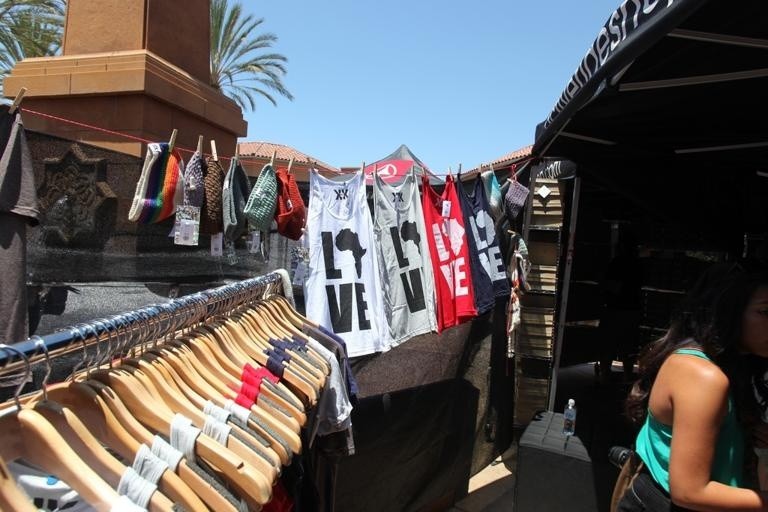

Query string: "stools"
[514, 408, 603, 512]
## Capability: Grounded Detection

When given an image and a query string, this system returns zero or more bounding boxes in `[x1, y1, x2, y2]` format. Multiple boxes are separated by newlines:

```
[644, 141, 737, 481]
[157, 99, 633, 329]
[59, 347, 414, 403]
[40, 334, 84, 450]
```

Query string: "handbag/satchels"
[617, 472, 686, 512]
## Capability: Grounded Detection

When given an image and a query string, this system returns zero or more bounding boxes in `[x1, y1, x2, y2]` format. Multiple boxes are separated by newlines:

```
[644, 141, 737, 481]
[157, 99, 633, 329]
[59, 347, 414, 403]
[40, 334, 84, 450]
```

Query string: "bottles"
[563, 399, 576, 437]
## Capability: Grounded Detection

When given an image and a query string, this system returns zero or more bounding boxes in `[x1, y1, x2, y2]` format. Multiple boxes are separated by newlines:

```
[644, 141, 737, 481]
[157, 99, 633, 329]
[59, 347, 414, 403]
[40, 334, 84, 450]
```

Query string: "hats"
[241, 163, 279, 234]
[182, 150, 204, 208]
[275, 166, 306, 241]
[220, 157, 253, 242]
[202, 154, 226, 236]
[127, 142, 184, 227]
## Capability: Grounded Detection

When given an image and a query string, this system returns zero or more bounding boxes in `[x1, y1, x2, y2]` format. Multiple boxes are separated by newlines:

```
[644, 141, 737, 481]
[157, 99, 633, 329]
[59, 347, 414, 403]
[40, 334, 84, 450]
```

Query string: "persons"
[615, 260, 768, 512]
[751, 370, 768, 491]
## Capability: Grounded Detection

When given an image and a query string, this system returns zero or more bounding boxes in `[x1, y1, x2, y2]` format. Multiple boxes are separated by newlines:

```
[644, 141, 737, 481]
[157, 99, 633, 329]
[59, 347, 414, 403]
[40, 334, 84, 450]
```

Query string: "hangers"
[0, 279, 345, 511]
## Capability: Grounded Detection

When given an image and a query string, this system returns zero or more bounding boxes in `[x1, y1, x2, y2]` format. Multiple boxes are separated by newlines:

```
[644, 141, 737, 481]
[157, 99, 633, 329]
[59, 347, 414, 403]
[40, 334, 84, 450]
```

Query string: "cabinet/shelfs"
[513, 174, 565, 427]
